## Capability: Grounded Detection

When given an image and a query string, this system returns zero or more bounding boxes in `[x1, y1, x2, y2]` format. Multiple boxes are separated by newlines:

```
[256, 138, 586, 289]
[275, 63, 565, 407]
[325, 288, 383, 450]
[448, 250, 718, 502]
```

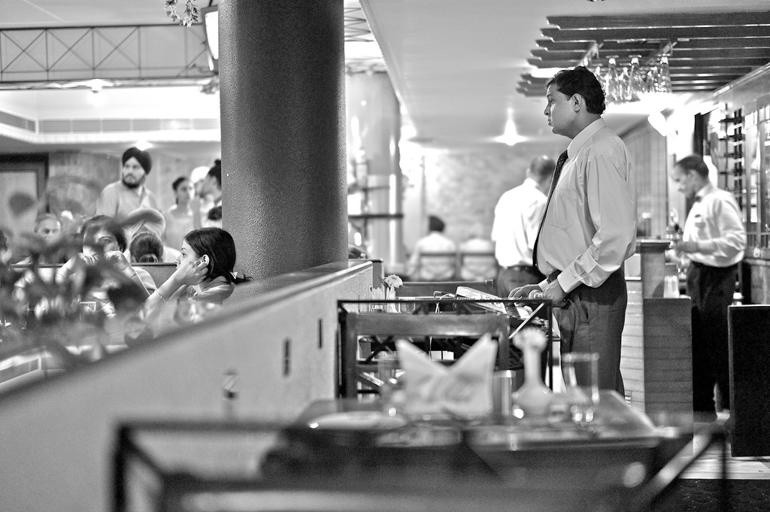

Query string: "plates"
[309, 408, 407, 434]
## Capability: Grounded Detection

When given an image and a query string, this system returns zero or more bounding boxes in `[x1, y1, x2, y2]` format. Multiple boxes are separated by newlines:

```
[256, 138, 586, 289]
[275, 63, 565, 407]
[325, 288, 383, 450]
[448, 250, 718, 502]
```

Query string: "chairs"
[104, 247, 734, 511]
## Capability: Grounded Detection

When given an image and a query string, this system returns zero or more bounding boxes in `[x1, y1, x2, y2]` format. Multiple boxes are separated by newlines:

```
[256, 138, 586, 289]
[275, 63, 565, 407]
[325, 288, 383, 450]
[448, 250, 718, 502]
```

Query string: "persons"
[0, 145, 253, 361]
[664, 153, 748, 423]
[508, 64, 637, 402]
[489, 154, 558, 391]
[406, 213, 455, 282]
[455, 224, 499, 282]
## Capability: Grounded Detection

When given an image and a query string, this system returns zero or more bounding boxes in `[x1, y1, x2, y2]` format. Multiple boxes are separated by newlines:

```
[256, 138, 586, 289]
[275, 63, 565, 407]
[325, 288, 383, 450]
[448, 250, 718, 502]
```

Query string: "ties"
[532, 149, 569, 268]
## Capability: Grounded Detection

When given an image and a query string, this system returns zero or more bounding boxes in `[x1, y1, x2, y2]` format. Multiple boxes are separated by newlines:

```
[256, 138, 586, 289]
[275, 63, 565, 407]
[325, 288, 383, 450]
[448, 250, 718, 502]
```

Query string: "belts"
[507, 264, 533, 273]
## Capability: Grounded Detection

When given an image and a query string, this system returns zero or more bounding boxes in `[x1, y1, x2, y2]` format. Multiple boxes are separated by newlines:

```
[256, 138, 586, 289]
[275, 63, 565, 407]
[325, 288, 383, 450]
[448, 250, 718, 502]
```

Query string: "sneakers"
[693, 410, 721, 423]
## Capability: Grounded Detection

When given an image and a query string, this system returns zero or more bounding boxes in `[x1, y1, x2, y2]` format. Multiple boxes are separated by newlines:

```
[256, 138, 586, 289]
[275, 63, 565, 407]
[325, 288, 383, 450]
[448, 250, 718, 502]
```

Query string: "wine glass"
[584, 48, 674, 105]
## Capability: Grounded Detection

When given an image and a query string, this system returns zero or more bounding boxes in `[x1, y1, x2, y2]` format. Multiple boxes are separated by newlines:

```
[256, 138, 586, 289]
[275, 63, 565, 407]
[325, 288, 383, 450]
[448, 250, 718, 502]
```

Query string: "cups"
[559, 351, 600, 429]
[491, 370, 515, 421]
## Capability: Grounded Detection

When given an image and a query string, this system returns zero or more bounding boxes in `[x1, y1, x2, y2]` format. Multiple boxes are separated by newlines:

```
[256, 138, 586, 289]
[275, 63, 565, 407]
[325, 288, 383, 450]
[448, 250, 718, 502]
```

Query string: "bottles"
[512, 329, 557, 420]
